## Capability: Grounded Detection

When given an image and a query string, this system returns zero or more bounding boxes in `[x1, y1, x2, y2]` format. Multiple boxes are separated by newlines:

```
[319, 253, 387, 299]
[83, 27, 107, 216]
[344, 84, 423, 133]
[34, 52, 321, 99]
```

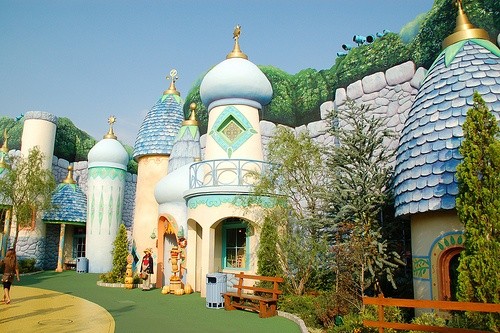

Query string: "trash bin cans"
[76, 257, 88, 274]
[205, 273, 227, 309]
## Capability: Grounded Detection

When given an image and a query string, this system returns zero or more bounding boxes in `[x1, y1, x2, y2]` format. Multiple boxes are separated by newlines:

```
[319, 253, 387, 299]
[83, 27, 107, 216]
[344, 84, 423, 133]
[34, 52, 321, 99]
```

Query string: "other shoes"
[0, 300, 6, 304]
[6, 299, 11, 304]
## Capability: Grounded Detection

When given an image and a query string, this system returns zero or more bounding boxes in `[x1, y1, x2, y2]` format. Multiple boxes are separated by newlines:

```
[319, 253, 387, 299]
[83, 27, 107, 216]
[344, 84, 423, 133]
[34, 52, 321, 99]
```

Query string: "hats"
[144, 248, 153, 254]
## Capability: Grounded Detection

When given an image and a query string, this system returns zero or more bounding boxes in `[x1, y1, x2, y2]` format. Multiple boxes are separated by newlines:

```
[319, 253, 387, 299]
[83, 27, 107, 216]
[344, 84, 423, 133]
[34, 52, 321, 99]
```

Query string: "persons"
[140, 248, 153, 290]
[0, 249, 19, 303]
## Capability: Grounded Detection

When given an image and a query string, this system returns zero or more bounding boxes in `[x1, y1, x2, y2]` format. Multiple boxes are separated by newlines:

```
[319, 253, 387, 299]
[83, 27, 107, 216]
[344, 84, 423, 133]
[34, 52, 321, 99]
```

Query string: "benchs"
[362, 297, 500, 333]
[221, 272, 285, 318]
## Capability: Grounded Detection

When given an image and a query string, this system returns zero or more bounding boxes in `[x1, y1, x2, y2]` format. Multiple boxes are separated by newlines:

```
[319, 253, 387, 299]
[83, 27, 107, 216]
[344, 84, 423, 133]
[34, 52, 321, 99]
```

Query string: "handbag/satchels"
[138, 270, 147, 279]
[1, 273, 12, 282]
